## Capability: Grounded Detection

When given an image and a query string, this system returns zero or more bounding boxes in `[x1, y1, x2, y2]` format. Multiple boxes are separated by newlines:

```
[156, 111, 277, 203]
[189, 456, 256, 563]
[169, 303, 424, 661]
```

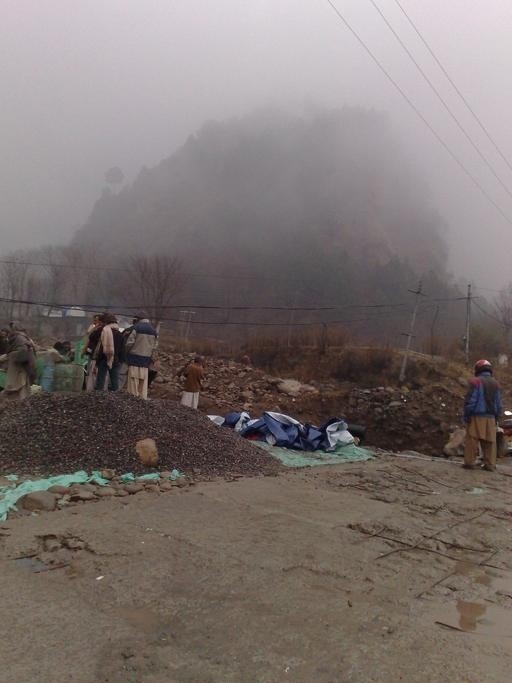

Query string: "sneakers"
[481, 464, 495, 471]
[462, 463, 474, 469]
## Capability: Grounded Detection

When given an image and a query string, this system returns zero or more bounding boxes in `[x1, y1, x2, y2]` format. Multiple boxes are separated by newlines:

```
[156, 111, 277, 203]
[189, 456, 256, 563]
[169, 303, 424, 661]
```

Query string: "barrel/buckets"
[53, 360, 88, 394]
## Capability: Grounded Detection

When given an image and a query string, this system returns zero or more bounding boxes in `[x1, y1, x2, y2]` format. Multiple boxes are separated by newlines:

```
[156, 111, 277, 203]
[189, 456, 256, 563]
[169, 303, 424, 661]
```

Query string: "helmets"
[474, 360, 492, 376]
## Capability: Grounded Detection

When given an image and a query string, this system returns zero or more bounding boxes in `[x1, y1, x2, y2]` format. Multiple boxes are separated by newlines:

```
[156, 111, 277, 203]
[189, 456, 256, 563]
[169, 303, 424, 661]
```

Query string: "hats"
[133, 313, 146, 319]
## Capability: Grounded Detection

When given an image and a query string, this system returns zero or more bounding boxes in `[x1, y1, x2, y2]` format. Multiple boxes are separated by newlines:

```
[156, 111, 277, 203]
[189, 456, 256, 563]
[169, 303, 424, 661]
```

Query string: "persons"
[181, 355, 207, 410]
[461, 359, 503, 472]
[0, 305, 163, 409]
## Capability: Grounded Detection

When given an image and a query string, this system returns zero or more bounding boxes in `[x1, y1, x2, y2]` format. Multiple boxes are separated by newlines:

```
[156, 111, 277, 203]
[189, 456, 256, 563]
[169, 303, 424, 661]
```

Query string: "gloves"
[464, 415, 471, 423]
[496, 416, 504, 426]
